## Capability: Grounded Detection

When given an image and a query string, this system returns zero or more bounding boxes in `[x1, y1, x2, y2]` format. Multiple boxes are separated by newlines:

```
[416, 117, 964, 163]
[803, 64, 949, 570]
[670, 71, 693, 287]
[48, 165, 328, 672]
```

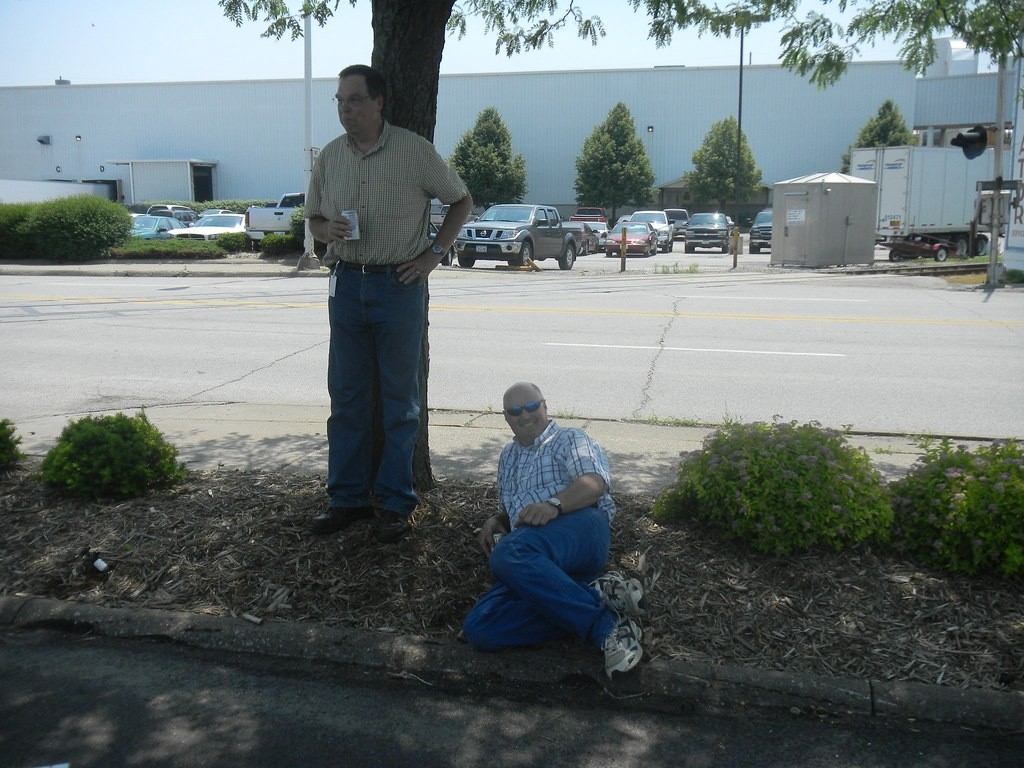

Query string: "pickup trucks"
[569, 207, 609, 223]
[430, 205, 478, 232]
[453, 203, 584, 270]
[244, 192, 305, 250]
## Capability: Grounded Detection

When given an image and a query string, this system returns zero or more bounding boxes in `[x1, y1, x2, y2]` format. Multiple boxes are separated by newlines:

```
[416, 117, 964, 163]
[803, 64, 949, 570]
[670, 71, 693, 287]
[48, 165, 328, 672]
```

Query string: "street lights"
[734, 11, 751, 268]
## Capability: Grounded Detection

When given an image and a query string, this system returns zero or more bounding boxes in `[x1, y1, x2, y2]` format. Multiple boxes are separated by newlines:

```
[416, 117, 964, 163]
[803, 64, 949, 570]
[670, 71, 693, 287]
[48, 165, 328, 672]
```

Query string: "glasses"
[332, 95, 370, 106]
[507, 399, 546, 416]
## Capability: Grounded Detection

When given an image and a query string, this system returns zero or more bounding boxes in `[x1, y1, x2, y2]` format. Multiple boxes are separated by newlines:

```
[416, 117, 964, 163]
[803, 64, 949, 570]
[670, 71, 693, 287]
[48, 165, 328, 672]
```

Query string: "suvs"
[623, 211, 676, 254]
[748, 210, 772, 254]
[681, 213, 730, 254]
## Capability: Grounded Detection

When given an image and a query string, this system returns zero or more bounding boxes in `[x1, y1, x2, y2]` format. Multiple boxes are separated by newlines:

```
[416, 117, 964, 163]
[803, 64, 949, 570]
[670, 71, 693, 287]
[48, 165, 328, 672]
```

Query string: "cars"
[127, 204, 246, 242]
[664, 209, 689, 236]
[603, 221, 660, 256]
[429, 221, 455, 267]
[584, 222, 612, 250]
[726, 216, 735, 236]
[561, 222, 601, 256]
[614, 215, 632, 226]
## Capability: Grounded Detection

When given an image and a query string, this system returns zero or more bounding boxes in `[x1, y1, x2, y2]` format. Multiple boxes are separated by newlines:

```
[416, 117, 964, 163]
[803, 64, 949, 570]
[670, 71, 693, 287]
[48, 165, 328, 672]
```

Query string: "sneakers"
[601, 611, 643, 682]
[311, 507, 359, 534]
[588, 570, 643, 628]
[376, 510, 409, 543]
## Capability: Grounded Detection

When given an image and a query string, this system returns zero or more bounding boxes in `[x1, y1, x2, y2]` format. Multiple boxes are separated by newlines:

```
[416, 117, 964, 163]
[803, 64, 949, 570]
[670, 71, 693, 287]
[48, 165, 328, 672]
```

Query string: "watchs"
[430, 243, 448, 256]
[544, 497, 562, 520]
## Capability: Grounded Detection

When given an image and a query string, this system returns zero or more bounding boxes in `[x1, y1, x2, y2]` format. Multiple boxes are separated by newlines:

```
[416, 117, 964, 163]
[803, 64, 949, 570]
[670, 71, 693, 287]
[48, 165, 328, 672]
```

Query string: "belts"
[334, 260, 399, 272]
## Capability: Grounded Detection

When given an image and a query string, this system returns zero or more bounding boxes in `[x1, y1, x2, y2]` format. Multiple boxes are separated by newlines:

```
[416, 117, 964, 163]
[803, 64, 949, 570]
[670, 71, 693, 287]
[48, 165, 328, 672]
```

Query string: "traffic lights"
[949, 126, 989, 160]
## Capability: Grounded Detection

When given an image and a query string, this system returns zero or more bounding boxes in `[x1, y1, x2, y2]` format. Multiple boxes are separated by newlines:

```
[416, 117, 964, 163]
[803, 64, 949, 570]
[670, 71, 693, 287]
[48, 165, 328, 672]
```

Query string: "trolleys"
[876, 233, 949, 262]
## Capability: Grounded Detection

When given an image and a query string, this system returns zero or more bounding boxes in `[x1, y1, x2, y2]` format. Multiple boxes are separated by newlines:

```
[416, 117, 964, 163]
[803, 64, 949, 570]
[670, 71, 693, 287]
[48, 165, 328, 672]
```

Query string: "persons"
[463, 381, 646, 682]
[303, 65, 472, 543]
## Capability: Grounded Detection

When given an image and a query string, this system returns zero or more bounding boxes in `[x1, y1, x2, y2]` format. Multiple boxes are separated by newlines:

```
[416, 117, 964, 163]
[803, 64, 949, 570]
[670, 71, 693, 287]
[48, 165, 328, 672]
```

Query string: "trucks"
[848, 145, 1011, 257]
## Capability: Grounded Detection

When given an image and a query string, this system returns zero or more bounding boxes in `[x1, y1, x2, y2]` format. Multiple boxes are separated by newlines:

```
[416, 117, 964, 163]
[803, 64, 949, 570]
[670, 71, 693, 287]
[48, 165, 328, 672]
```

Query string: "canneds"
[490, 533, 505, 551]
[341, 210, 360, 240]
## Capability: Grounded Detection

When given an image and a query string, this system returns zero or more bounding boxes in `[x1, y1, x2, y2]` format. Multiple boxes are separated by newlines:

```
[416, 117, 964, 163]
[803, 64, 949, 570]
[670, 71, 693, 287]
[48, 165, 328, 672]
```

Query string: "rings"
[416, 271, 420, 277]
